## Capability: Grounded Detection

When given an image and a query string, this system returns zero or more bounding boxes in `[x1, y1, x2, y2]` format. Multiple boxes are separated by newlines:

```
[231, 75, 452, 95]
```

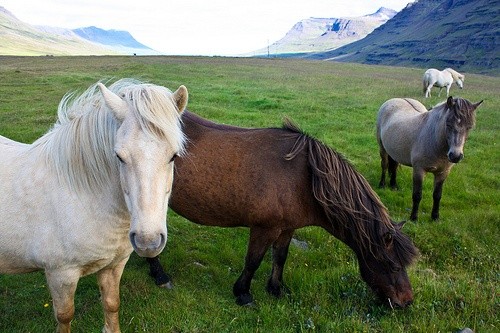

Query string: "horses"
[375, 94, 484, 224]
[423, 67, 465, 97]
[0, 77, 188, 333]
[146, 110, 423, 308]
